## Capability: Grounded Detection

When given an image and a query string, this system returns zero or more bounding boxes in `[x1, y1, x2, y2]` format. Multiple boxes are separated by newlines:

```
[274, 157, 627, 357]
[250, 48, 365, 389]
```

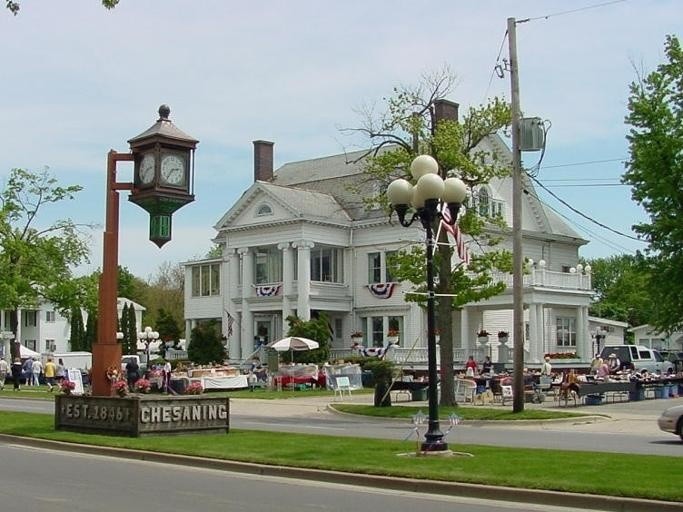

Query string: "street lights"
[388, 155, 467, 459]
[139, 326, 160, 371]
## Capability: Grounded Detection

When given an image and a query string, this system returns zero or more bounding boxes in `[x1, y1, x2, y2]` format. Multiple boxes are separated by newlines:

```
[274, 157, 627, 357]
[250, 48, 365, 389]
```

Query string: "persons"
[126, 357, 140, 392]
[249, 357, 264, 374]
[162, 357, 171, 395]
[0, 357, 66, 392]
[466, 354, 620, 405]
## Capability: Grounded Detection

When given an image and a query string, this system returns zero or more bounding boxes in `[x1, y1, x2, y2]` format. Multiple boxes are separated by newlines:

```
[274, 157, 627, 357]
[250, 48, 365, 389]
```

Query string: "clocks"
[159, 153, 184, 184]
[138, 153, 155, 185]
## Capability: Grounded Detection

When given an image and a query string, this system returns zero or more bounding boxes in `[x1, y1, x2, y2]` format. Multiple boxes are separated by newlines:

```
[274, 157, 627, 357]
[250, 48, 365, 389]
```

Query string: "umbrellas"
[267, 337, 319, 362]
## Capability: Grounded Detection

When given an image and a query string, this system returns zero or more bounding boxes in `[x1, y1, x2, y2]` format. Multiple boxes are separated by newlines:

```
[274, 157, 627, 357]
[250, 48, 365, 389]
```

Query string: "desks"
[575, 377, 683, 406]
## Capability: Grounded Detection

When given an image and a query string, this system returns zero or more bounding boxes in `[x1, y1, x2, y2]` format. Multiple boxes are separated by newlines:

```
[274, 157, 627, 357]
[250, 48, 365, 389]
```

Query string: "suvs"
[600, 344, 673, 375]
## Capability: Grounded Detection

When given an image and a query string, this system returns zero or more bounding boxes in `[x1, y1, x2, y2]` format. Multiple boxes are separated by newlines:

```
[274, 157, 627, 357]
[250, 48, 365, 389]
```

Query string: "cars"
[657, 404, 683, 440]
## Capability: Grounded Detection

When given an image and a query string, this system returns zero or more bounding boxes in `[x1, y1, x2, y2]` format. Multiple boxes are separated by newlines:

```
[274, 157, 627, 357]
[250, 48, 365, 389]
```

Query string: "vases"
[435, 335, 440, 348]
[498, 337, 508, 347]
[388, 336, 399, 347]
[478, 336, 489, 348]
[352, 337, 362, 346]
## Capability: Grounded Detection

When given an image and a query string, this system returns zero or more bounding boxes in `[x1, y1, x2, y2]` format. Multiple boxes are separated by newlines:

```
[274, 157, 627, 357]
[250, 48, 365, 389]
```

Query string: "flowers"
[476, 330, 492, 338]
[497, 331, 510, 338]
[434, 328, 442, 335]
[351, 331, 363, 338]
[387, 329, 399, 337]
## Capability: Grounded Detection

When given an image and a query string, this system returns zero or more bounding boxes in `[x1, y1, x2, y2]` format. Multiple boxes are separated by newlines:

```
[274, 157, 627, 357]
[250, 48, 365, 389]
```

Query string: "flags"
[443, 206, 470, 264]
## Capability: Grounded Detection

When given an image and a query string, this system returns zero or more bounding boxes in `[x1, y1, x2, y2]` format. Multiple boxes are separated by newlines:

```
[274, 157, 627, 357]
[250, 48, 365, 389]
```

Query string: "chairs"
[454, 378, 513, 406]
